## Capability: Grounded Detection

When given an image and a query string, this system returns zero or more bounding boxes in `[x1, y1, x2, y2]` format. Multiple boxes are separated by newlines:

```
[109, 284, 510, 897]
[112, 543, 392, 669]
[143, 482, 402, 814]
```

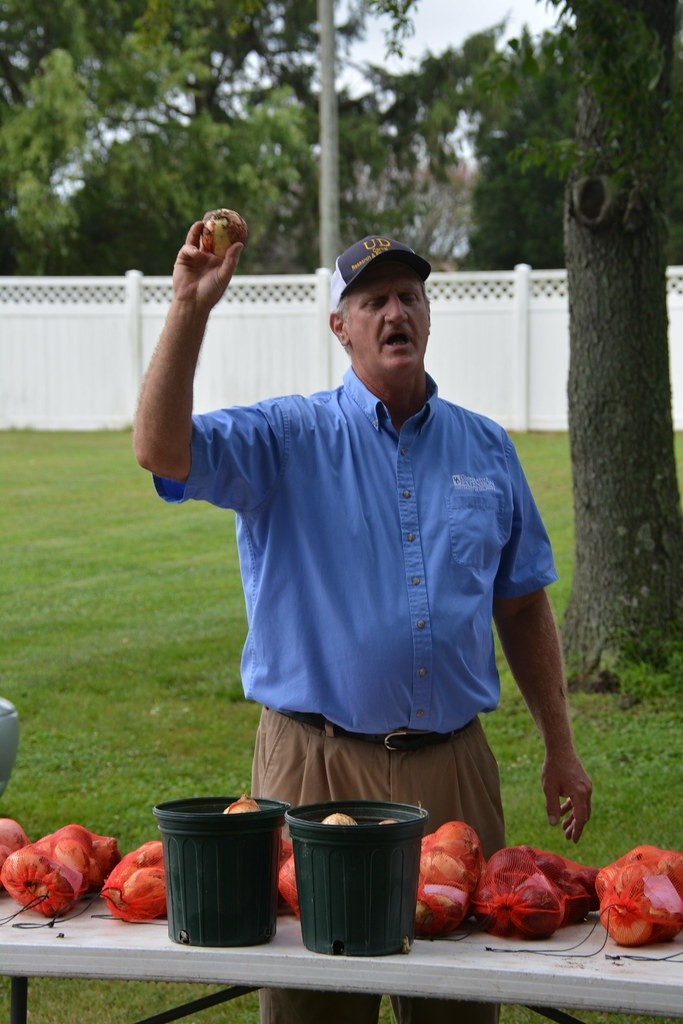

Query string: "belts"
[278, 710, 477, 749]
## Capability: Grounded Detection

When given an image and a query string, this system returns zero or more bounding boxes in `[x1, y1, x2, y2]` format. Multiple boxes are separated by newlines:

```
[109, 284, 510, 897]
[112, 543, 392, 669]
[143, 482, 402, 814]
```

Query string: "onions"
[1, 796, 683, 942]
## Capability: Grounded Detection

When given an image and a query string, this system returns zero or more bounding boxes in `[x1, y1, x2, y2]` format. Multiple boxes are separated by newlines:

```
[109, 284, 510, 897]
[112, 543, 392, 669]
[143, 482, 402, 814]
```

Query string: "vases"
[151, 797, 291, 947]
[283, 800, 430, 957]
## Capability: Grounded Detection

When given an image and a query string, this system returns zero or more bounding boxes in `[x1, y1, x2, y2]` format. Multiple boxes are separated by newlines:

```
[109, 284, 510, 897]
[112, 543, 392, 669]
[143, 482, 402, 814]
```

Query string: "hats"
[330, 236, 431, 312]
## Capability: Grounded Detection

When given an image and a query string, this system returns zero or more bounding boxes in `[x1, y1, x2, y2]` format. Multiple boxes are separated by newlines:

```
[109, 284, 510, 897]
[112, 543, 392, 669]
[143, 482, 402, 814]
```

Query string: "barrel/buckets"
[151, 794, 290, 947]
[285, 800, 429, 959]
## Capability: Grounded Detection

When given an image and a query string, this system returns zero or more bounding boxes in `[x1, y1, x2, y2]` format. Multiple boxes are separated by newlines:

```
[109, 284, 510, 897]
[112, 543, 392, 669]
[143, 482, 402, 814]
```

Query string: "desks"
[0, 896, 683, 1024]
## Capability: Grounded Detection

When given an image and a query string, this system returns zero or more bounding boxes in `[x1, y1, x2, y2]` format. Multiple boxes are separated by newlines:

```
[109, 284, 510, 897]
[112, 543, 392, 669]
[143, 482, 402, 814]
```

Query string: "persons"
[133, 210, 592, 1024]
